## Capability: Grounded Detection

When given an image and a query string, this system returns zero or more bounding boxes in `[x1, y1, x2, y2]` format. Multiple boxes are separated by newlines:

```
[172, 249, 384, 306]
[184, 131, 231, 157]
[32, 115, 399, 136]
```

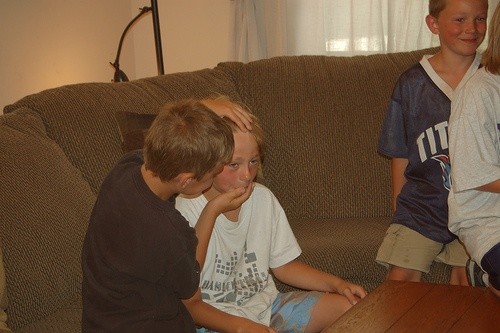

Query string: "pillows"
[115, 110, 158, 153]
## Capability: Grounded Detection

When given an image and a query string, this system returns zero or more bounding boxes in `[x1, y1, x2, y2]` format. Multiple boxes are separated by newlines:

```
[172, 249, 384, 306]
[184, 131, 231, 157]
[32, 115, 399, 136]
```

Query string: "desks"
[321, 279, 500, 333]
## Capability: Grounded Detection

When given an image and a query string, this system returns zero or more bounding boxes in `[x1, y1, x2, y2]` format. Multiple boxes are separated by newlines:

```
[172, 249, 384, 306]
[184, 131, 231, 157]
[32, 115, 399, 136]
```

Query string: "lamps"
[109, 0, 164, 82]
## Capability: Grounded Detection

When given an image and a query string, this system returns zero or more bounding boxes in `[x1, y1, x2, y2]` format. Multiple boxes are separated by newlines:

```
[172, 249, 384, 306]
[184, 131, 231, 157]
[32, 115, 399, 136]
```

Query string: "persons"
[376, 0, 488, 289]
[80, 95, 254, 333]
[447, 0, 500, 297]
[174, 112, 367, 333]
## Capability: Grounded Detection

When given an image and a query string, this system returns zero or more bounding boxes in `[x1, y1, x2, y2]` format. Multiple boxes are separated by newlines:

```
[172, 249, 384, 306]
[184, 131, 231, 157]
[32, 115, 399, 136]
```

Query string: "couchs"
[0, 45, 453, 333]
